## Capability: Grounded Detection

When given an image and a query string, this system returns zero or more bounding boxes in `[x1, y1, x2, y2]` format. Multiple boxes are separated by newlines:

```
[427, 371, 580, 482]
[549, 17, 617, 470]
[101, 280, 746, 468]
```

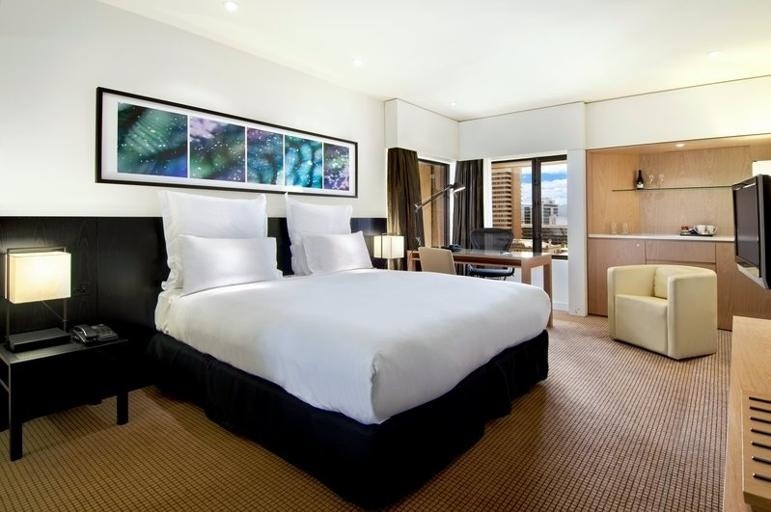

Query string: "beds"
[146, 267, 552, 512]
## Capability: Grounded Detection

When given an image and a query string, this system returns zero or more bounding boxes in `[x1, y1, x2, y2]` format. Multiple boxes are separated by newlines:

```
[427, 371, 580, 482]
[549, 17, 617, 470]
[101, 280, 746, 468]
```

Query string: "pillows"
[163, 191, 269, 290]
[181, 236, 278, 296]
[285, 194, 352, 276]
[304, 230, 372, 277]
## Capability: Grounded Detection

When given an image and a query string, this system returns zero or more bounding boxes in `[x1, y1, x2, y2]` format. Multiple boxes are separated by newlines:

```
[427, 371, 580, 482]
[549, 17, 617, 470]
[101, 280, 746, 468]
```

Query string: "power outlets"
[73, 285, 90, 297]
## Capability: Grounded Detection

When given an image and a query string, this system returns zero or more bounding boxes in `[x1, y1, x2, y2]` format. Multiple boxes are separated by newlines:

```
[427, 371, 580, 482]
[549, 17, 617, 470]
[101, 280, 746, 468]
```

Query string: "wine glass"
[645, 175, 656, 189]
[655, 172, 667, 190]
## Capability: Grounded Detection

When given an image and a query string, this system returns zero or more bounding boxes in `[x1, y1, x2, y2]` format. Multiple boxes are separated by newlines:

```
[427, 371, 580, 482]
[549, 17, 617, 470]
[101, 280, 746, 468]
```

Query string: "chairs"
[419, 248, 456, 275]
[468, 229, 514, 280]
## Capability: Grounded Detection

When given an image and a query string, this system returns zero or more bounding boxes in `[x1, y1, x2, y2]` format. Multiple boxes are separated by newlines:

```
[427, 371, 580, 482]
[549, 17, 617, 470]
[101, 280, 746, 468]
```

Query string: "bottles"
[636, 170, 644, 190]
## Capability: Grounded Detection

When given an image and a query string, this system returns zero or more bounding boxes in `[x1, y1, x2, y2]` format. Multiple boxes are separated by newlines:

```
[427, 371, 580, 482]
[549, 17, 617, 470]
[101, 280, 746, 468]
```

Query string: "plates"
[697, 231, 713, 235]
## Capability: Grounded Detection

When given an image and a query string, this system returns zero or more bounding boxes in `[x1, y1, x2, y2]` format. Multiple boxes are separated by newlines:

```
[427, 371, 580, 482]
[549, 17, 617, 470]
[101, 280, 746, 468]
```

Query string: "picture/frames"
[99, 87, 359, 198]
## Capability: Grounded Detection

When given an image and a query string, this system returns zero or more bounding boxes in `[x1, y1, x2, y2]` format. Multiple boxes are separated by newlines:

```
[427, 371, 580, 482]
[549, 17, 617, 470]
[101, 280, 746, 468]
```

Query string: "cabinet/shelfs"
[585, 134, 771, 332]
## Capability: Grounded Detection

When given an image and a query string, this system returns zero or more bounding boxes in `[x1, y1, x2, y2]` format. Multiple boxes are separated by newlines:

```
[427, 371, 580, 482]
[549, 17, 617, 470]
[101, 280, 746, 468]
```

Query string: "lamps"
[413, 182, 466, 250]
[374, 233, 404, 270]
[2, 246, 72, 352]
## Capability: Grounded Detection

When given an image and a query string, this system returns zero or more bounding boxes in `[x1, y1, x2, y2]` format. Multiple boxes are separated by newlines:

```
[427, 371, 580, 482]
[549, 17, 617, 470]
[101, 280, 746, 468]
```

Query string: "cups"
[694, 225, 705, 233]
[707, 225, 717, 233]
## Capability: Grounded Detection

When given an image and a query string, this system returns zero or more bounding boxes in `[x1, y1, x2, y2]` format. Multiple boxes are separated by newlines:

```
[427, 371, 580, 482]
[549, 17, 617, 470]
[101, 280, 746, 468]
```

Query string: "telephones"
[441, 244, 461, 252]
[71, 324, 117, 347]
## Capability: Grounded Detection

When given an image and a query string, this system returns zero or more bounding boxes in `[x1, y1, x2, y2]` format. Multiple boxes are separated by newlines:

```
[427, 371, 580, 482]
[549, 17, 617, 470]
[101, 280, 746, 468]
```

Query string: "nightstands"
[1, 323, 129, 462]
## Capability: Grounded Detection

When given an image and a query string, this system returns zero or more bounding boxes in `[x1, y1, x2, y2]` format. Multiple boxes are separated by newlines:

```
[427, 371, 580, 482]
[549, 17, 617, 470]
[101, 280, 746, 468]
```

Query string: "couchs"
[607, 265, 718, 360]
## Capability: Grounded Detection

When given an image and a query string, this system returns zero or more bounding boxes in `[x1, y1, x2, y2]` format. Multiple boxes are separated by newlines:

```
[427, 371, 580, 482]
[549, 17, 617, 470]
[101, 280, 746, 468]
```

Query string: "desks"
[720, 315, 771, 510]
[407, 246, 554, 328]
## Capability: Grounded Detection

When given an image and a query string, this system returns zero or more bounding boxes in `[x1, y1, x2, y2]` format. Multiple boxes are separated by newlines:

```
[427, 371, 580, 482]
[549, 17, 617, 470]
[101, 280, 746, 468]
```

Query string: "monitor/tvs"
[732, 175, 767, 283]
